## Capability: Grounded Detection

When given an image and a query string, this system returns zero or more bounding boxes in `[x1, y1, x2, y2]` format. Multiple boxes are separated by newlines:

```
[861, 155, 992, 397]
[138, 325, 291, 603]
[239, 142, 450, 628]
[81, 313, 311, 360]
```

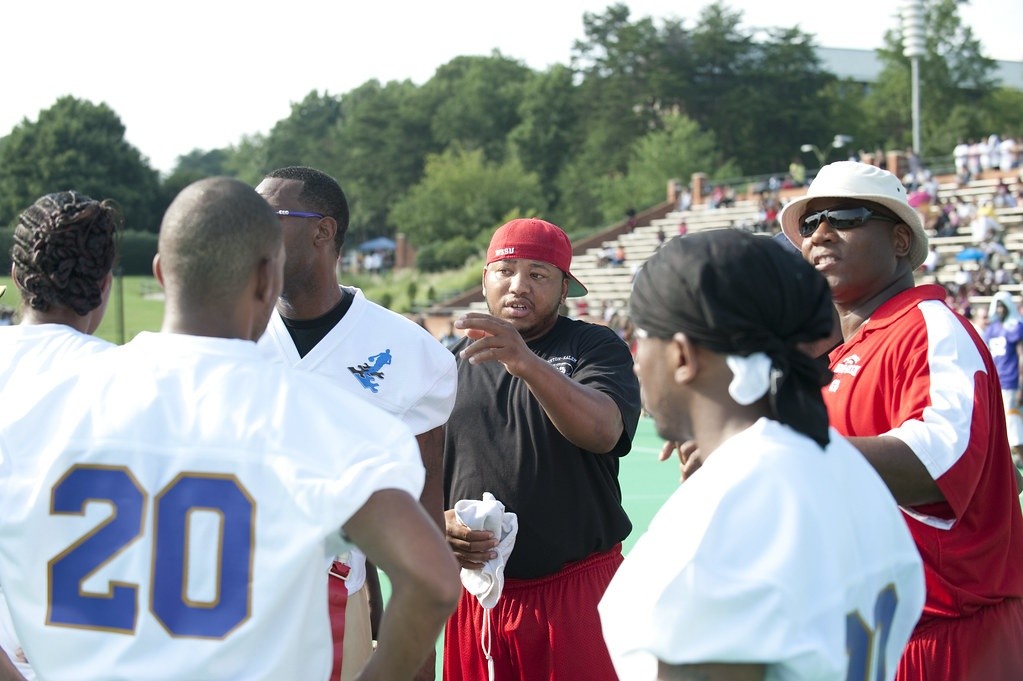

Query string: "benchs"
[441, 176, 1023, 348]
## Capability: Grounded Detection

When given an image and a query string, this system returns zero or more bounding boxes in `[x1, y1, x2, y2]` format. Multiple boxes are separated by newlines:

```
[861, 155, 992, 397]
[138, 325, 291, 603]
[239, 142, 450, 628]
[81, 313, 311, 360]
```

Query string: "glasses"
[798, 203, 896, 238]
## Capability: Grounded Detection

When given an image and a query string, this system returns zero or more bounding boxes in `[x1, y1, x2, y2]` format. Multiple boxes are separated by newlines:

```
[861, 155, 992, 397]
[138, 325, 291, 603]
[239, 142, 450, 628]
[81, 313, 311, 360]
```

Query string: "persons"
[1, 177, 460, 681]
[1, 191, 122, 395]
[559, 129, 1023, 477]
[363, 251, 384, 276]
[658, 160, 1022, 681]
[595, 226, 928, 680]
[253, 166, 460, 681]
[436, 219, 642, 680]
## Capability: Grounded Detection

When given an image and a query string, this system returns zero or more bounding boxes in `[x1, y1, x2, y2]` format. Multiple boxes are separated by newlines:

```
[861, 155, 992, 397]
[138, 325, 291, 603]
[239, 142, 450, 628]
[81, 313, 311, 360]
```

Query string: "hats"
[485, 218, 588, 298]
[780, 160, 929, 272]
